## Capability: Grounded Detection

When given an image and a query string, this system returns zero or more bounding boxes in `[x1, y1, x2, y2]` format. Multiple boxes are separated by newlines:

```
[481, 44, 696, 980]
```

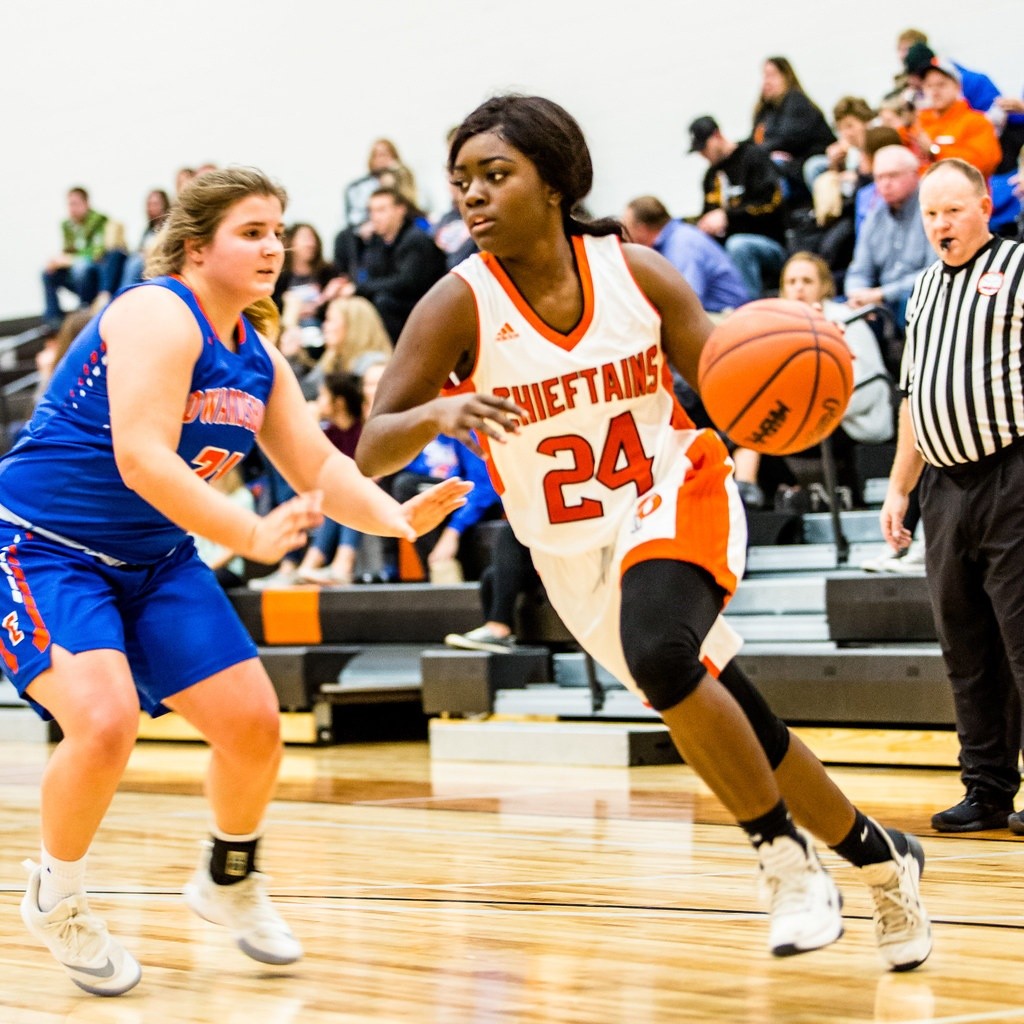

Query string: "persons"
[876, 157, 1024, 836]
[35, 26, 1020, 654]
[351, 96, 936, 974]
[1, 166, 475, 1003]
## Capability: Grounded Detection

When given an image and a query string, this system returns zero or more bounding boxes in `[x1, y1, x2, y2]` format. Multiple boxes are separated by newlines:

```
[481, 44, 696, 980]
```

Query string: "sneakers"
[445, 626, 515, 655]
[755, 828, 844, 957]
[19, 859, 142, 996]
[248, 567, 353, 590]
[1008, 809, 1024, 835]
[186, 840, 303, 965]
[932, 798, 1016, 832]
[857, 815, 934, 972]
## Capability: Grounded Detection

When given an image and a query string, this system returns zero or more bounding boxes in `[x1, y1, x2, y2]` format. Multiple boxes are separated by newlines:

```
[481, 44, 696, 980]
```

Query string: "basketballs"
[695, 296, 855, 458]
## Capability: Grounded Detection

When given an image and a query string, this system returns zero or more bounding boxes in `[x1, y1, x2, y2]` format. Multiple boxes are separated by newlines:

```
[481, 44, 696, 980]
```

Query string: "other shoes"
[784, 485, 810, 516]
[731, 478, 763, 509]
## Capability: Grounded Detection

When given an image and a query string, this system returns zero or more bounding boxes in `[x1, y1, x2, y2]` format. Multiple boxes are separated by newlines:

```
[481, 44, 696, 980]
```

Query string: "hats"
[919, 56, 963, 85]
[690, 116, 718, 153]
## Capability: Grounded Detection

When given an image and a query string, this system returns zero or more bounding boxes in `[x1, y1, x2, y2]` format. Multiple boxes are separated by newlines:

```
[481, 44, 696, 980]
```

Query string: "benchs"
[686, 270, 961, 727]
[222, 516, 577, 715]
